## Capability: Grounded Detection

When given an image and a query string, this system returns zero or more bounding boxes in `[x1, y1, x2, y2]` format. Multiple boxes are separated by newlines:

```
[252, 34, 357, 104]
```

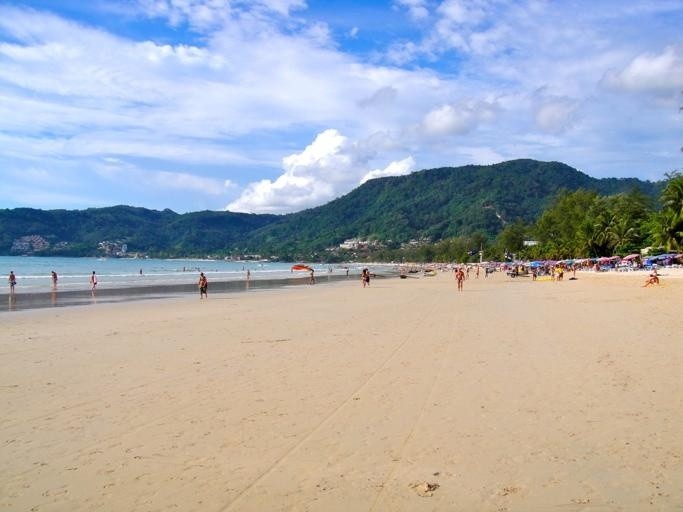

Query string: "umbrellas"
[498, 251, 683, 282]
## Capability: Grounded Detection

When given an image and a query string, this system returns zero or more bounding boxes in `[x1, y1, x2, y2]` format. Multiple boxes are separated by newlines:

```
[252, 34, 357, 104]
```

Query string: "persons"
[643, 274, 659, 287]
[362, 269, 369, 287]
[454, 268, 465, 293]
[8, 271, 15, 292]
[50, 270, 57, 286]
[89, 270, 96, 289]
[361, 268, 368, 288]
[475, 266, 479, 277]
[198, 272, 207, 299]
[310, 270, 314, 281]
[246, 269, 250, 279]
